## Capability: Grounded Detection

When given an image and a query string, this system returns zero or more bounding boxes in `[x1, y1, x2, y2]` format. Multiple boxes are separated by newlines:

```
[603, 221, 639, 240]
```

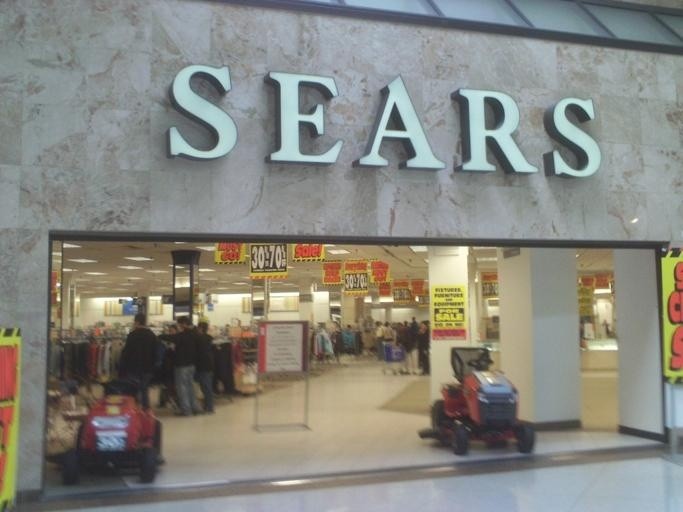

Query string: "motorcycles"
[73, 372, 165, 483]
[419, 346, 534, 453]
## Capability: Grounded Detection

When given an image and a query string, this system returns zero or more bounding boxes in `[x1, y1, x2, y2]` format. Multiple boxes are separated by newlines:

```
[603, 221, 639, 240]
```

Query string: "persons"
[168, 317, 204, 416]
[601, 319, 611, 335]
[193, 322, 219, 411]
[119, 313, 164, 422]
[156, 325, 178, 407]
[329, 316, 430, 378]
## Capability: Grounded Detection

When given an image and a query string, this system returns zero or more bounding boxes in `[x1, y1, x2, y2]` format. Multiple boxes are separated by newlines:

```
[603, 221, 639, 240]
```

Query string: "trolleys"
[378, 338, 415, 375]
